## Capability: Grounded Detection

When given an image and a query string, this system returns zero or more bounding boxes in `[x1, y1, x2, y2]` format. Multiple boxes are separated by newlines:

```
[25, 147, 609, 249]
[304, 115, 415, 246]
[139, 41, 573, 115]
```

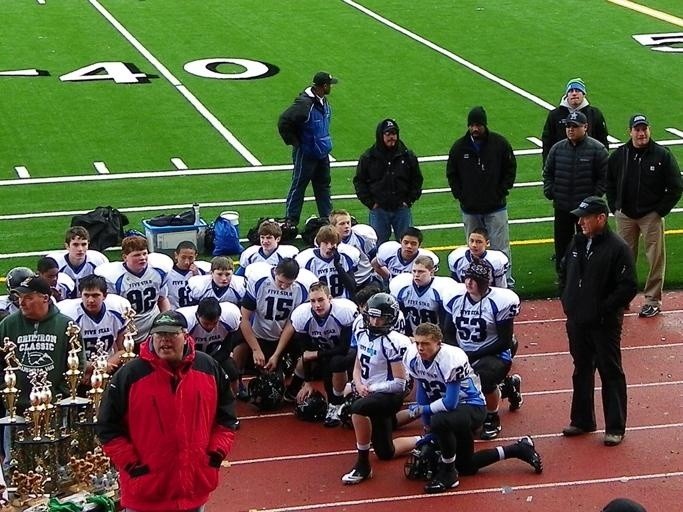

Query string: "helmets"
[338, 392, 358, 428]
[5, 266, 35, 297]
[404, 442, 439, 481]
[296, 390, 328, 423]
[362, 292, 399, 336]
[250, 368, 286, 412]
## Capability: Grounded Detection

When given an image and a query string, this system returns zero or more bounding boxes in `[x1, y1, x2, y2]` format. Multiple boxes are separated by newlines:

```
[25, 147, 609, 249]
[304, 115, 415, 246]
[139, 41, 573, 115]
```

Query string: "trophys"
[0, 304, 138, 512]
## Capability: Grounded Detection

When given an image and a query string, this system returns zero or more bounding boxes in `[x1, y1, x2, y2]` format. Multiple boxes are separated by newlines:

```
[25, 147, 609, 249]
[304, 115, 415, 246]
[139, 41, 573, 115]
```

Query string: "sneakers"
[324, 401, 344, 426]
[506, 372, 523, 412]
[604, 433, 625, 445]
[238, 381, 252, 401]
[639, 304, 661, 318]
[424, 473, 462, 495]
[563, 424, 596, 436]
[519, 434, 543, 473]
[340, 462, 372, 482]
[479, 414, 502, 439]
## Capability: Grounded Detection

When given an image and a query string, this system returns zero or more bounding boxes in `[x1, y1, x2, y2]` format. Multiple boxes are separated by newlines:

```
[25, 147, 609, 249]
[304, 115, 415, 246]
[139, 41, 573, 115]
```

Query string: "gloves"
[408, 404, 423, 419]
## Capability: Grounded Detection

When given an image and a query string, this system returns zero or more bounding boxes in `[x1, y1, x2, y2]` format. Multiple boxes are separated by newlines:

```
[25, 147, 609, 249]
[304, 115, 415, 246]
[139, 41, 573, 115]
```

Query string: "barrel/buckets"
[219, 210, 240, 238]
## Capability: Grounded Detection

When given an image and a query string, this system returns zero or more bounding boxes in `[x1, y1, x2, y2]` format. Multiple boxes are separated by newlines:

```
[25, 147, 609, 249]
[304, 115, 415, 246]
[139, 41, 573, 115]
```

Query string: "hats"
[570, 196, 610, 217]
[382, 121, 399, 132]
[464, 259, 491, 294]
[629, 113, 650, 128]
[11, 274, 53, 297]
[559, 111, 588, 126]
[149, 310, 189, 335]
[312, 73, 337, 84]
[468, 105, 487, 126]
[565, 77, 587, 97]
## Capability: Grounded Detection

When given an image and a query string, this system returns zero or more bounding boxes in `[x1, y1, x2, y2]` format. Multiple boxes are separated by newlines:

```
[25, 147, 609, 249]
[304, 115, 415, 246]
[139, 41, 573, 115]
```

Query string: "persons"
[606, 114, 683, 318]
[93, 209, 543, 512]
[558, 196, 639, 446]
[541, 112, 610, 295]
[1, 226, 130, 481]
[446, 106, 516, 290]
[353, 119, 425, 245]
[540, 78, 610, 174]
[277, 72, 338, 225]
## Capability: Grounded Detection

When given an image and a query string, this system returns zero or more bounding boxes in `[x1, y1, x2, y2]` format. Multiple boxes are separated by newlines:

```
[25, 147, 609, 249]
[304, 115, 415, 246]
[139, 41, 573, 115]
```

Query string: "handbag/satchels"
[70, 206, 147, 252]
[303, 214, 356, 244]
[203, 217, 246, 256]
[248, 215, 297, 245]
